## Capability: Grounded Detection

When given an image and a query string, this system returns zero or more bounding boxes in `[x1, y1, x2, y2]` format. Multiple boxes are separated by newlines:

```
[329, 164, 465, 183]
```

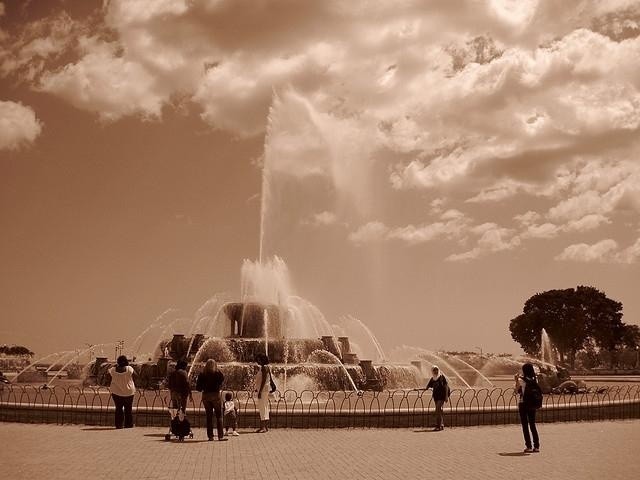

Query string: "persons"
[253, 351, 277, 432]
[513, 363, 543, 453]
[196, 358, 229, 441]
[424, 366, 449, 429]
[105, 355, 139, 429]
[223, 392, 239, 436]
[167, 358, 194, 428]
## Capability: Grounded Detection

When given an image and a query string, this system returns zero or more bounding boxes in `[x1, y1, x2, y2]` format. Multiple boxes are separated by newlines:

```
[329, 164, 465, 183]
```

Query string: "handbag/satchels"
[269, 378, 276, 393]
[443, 385, 451, 398]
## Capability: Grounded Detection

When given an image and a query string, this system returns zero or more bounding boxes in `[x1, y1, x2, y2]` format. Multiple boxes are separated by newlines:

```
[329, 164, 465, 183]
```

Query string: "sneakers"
[533, 447, 540, 452]
[209, 438, 213, 441]
[219, 438, 228, 441]
[256, 428, 269, 433]
[224, 431, 229, 436]
[232, 431, 239, 436]
[524, 447, 533, 453]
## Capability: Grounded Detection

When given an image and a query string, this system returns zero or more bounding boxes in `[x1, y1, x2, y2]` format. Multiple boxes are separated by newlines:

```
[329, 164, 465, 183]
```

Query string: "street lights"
[476, 346, 483, 360]
[85, 343, 95, 362]
[115, 340, 125, 357]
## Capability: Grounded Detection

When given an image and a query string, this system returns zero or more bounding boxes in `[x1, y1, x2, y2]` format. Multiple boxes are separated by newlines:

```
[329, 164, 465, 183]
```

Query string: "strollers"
[164, 397, 194, 443]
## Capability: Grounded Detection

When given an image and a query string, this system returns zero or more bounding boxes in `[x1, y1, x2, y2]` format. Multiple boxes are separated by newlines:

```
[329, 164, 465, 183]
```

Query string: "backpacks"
[520, 376, 543, 409]
